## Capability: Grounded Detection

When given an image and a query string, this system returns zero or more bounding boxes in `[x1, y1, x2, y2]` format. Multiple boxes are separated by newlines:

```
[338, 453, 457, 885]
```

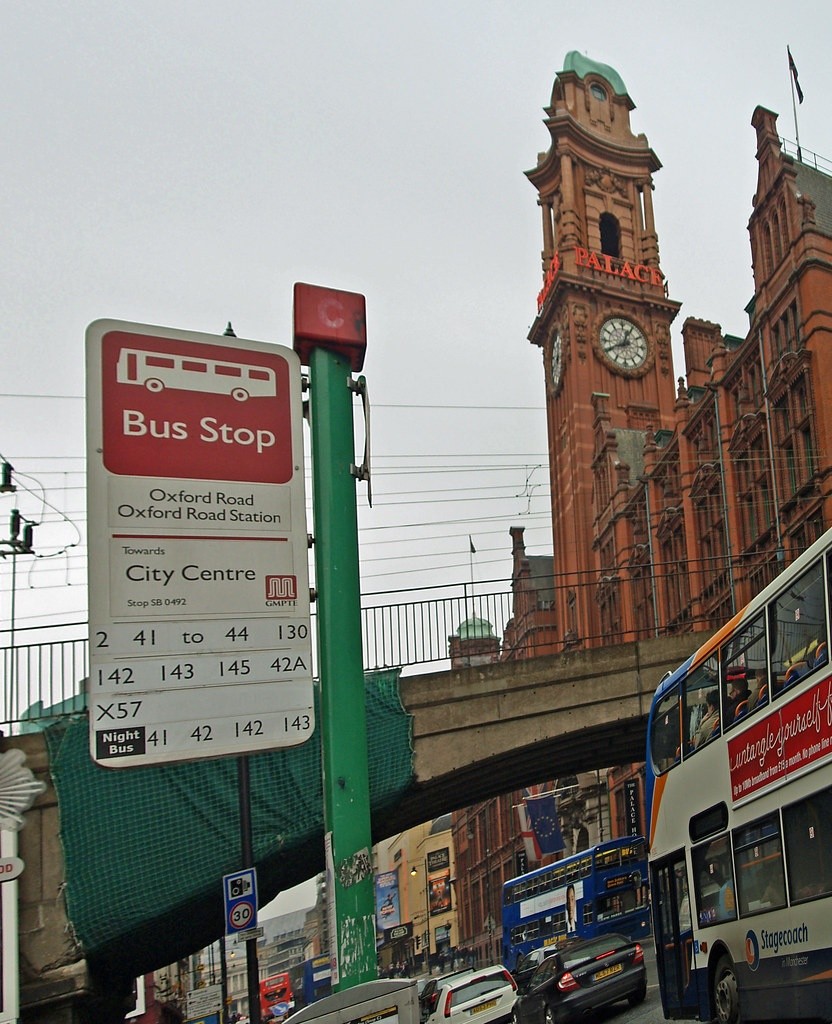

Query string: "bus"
[259, 972, 293, 1020]
[271, 950, 330, 1011]
[496, 836, 645, 976]
[638, 525, 832, 1024]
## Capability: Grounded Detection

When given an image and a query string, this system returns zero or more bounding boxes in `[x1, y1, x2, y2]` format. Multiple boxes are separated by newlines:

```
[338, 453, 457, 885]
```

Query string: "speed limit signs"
[222, 867, 258, 936]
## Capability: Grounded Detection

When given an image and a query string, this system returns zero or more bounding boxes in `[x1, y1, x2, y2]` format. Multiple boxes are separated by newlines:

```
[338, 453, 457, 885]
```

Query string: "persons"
[748, 668, 768, 712]
[566, 885, 578, 932]
[723, 678, 752, 728]
[693, 689, 720, 749]
[378, 945, 475, 979]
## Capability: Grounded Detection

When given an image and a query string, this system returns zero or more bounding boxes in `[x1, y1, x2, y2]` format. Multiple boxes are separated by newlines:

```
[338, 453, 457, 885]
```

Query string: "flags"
[518, 805, 545, 860]
[788, 50, 803, 104]
[526, 796, 566, 854]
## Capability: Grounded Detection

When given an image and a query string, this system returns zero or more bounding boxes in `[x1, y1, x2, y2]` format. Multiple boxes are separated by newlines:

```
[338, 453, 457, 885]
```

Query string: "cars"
[508, 936, 584, 997]
[510, 934, 648, 1024]
[418, 965, 520, 1023]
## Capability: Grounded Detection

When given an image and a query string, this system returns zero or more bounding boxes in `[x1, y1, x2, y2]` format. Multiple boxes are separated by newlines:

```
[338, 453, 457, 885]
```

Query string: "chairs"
[675, 641, 828, 757]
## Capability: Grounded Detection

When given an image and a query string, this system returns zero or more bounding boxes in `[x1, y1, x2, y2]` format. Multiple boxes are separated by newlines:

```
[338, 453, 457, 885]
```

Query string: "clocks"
[599, 316, 650, 371]
[551, 336, 564, 388]
